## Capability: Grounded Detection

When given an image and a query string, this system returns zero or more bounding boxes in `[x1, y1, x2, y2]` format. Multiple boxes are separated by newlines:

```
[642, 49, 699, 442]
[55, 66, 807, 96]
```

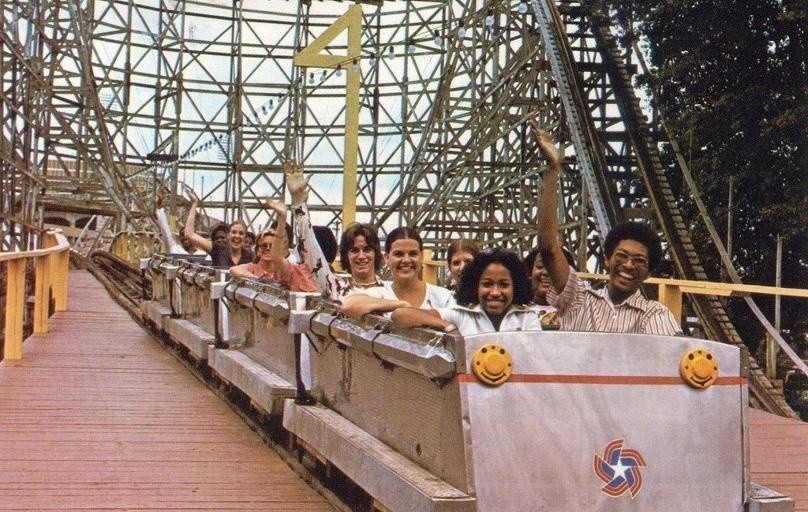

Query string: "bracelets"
[443, 324, 458, 332]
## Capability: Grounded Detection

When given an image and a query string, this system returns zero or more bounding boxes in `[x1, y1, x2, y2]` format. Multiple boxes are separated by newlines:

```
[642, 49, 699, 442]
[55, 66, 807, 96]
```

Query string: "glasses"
[615, 251, 649, 267]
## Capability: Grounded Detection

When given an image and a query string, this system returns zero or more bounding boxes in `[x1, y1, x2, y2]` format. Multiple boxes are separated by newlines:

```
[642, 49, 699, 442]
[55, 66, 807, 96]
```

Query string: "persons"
[267, 197, 338, 292]
[444, 238, 479, 291]
[391, 247, 557, 337]
[527, 113, 686, 338]
[522, 245, 576, 306]
[283, 158, 389, 302]
[156, 189, 256, 268]
[229, 220, 297, 283]
[339, 227, 452, 319]
